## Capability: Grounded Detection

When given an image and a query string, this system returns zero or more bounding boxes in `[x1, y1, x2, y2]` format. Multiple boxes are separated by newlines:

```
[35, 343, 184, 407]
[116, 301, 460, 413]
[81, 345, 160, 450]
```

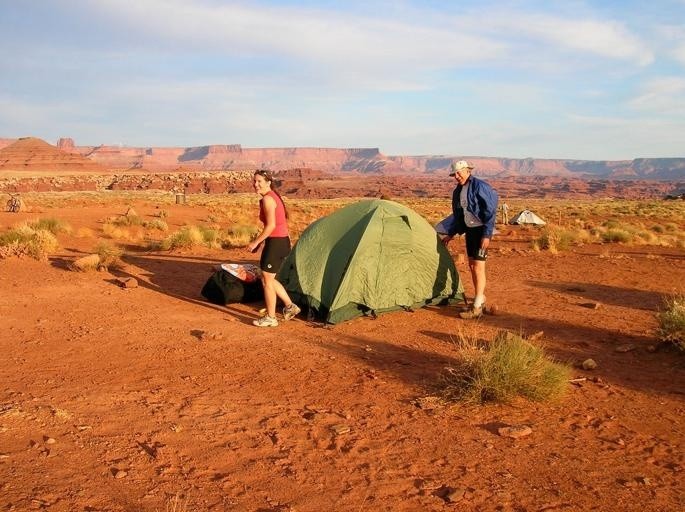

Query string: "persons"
[500, 201, 510, 225]
[244, 169, 303, 328]
[434, 160, 499, 319]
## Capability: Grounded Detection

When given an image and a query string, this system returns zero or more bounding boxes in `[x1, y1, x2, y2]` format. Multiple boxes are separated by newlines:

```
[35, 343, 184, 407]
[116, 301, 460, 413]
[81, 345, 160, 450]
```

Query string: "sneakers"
[280, 302, 301, 322]
[459, 307, 482, 319]
[253, 314, 279, 327]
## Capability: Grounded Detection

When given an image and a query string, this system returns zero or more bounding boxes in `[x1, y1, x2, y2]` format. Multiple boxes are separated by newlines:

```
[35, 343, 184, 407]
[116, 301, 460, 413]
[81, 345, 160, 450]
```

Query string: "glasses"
[256, 169, 268, 177]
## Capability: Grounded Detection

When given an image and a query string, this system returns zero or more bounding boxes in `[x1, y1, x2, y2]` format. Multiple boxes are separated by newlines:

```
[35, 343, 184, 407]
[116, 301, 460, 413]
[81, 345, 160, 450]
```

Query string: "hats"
[449, 161, 475, 177]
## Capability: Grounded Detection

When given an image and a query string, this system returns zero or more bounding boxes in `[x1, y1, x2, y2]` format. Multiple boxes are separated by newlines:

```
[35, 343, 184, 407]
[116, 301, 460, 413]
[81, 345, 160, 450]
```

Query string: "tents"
[258, 197, 469, 327]
[509, 208, 548, 226]
[434, 213, 499, 237]
